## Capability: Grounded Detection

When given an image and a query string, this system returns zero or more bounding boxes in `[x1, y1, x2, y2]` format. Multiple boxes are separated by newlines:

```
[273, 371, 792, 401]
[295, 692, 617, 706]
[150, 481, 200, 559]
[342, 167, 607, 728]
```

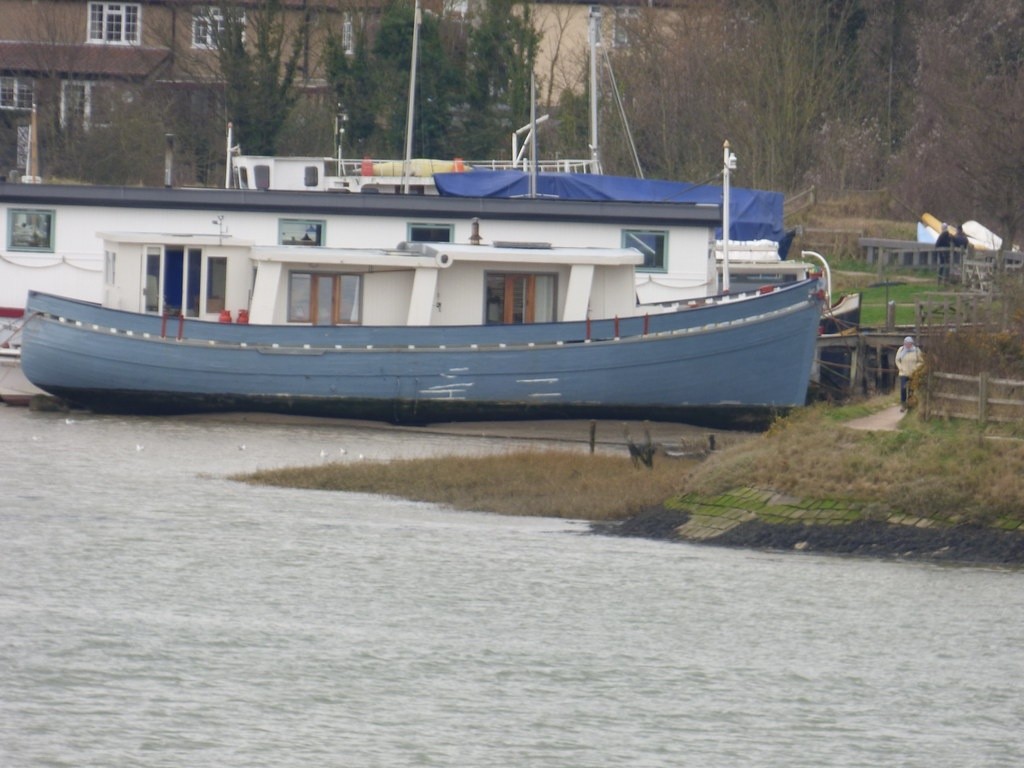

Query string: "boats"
[19, 138, 828, 433]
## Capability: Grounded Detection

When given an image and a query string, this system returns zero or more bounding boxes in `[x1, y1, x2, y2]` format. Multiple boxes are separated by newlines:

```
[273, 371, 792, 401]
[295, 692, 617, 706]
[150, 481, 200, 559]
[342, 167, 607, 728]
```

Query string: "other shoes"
[900, 401, 906, 412]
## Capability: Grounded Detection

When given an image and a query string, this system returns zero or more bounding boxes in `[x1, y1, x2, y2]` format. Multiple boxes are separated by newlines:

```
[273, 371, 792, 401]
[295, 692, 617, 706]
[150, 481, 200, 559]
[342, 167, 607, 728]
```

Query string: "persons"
[896, 338, 926, 412]
[934, 223, 969, 283]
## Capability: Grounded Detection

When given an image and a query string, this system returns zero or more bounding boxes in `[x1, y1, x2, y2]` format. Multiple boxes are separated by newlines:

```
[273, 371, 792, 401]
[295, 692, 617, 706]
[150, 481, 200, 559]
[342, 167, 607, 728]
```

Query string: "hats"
[904, 336, 913, 343]
